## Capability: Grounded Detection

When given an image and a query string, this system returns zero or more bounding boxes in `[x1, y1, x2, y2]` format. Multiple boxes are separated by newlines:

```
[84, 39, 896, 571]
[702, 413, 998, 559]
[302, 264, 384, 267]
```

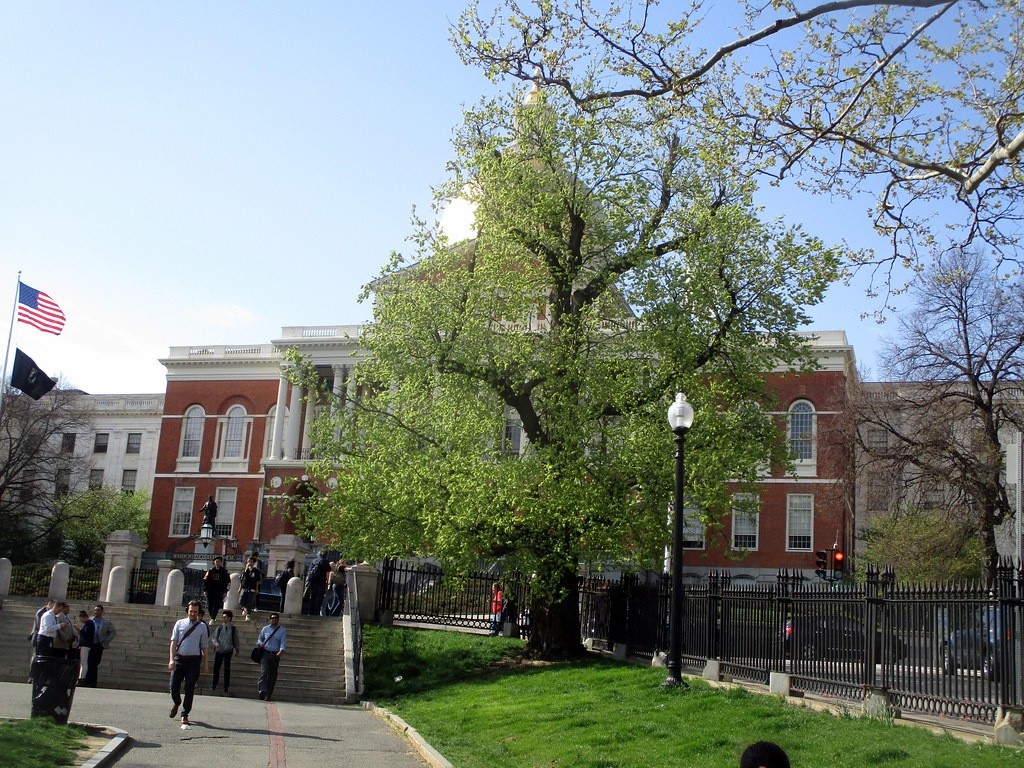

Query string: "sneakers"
[209, 618, 216, 625]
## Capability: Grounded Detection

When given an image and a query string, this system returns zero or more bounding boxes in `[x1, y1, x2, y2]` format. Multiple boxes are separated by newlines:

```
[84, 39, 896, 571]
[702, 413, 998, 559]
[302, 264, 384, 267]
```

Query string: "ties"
[55, 619, 61, 640]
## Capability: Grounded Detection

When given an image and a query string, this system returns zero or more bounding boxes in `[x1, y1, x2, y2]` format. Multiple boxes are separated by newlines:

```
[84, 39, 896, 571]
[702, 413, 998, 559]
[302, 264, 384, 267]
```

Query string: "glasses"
[270, 617, 279, 620]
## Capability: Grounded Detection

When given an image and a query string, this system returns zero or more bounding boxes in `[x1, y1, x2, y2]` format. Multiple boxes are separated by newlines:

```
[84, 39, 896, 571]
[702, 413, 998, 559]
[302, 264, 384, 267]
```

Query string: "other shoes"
[75, 680, 84, 687]
[211, 685, 216, 691]
[224, 689, 232, 695]
[259, 690, 272, 702]
[245, 615, 250, 621]
[242, 610, 248, 616]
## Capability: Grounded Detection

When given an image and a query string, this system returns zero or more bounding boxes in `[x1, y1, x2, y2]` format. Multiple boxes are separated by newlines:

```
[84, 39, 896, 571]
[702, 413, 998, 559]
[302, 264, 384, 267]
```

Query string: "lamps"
[198, 516, 214, 549]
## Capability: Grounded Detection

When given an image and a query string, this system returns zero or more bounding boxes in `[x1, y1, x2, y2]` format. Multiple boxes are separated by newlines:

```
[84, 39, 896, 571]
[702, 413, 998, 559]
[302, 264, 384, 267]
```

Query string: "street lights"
[658, 391, 695, 692]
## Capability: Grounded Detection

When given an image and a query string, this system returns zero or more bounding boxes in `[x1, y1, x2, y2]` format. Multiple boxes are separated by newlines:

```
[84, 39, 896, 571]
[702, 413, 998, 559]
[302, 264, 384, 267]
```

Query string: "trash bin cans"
[32, 658, 80, 725]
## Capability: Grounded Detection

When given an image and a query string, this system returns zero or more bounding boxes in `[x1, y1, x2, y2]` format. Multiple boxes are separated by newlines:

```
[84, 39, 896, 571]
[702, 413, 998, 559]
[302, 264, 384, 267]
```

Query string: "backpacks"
[275, 571, 285, 588]
[307, 559, 322, 584]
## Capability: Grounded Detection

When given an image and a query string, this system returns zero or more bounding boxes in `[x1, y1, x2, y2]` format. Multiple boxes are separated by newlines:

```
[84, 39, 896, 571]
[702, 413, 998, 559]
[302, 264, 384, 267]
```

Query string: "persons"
[74, 610, 95, 687]
[27, 600, 56, 684]
[304, 549, 346, 617]
[203, 556, 231, 625]
[86, 604, 116, 687]
[487, 582, 506, 634]
[54, 603, 74, 648]
[168, 600, 210, 723]
[238, 551, 264, 622]
[256, 613, 287, 701]
[279, 558, 296, 613]
[212, 611, 240, 692]
[199, 496, 218, 526]
[36, 601, 66, 657]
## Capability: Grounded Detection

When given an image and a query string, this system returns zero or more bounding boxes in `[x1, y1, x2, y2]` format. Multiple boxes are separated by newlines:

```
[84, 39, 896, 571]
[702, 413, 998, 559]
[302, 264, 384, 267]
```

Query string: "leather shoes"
[170, 705, 178, 719]
[181, 716, 190, 724]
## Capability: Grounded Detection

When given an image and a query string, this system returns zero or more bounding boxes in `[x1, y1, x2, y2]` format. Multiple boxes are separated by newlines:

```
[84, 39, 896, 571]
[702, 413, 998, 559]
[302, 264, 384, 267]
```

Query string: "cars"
[256, 577, 283, 614]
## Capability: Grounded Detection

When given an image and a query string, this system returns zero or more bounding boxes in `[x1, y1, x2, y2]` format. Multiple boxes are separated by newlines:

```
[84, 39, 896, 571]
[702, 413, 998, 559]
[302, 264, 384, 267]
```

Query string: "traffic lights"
[832, 549, 845, 579]
[814, 551, 827, 579]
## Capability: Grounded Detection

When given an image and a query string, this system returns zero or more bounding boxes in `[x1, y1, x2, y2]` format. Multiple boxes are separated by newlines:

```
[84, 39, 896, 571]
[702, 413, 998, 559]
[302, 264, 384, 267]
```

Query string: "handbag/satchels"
[250, 648, 262, 664]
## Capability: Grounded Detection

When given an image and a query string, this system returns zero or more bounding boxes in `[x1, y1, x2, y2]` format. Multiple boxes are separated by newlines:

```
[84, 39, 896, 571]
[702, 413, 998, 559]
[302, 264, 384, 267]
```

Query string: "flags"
[18, 281, 66, 335]
[11, 348, 57, 401]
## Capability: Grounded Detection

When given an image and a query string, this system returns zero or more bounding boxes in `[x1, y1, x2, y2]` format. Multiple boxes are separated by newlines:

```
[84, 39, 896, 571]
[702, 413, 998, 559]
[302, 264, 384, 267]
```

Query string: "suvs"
[939, 605, 1005, 682]
[778, 614, 908, 665]
